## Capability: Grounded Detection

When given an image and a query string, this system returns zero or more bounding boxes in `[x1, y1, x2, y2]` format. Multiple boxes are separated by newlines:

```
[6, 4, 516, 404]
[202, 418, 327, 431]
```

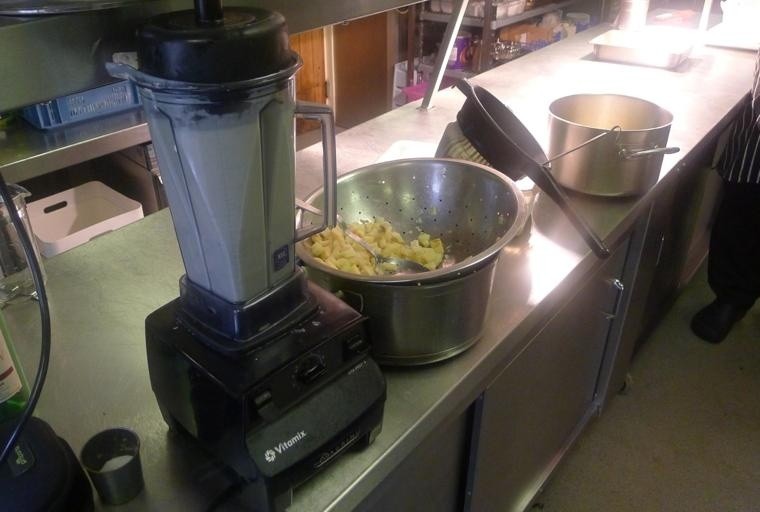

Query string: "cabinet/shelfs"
[418, 0, 607, 80]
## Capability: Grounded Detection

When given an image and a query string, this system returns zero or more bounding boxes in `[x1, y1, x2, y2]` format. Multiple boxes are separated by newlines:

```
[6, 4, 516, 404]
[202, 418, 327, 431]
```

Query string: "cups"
[566, 12, 590, 33]
[606, 0, 652, 33]
[81, 427, 148, 506]
[491, 38, 522, 64]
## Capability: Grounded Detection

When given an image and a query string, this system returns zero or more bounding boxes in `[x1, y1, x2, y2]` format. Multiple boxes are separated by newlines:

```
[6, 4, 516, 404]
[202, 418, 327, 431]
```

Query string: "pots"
[546, 93, 682, 200]
[294, 253, 501, 370]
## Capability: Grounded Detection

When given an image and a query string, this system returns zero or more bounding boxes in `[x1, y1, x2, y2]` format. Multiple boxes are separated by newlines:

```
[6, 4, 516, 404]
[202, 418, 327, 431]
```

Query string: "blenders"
[123, 1, 388, 510]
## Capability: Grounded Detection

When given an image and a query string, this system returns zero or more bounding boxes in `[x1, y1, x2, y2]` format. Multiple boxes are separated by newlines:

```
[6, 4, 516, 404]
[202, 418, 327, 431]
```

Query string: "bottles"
[0, 308, 33, 421]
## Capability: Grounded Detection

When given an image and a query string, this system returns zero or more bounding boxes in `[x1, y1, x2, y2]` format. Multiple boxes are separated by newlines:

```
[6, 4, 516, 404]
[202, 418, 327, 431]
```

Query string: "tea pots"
[459, 37, 494, 75]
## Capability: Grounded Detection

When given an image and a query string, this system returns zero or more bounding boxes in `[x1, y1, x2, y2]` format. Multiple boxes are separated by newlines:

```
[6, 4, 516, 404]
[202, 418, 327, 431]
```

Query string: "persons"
[690, 40, 759, 345]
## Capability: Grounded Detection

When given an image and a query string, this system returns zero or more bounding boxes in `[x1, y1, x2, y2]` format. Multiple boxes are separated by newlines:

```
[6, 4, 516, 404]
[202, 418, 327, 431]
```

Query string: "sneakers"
[687, 289, 760, 345]
